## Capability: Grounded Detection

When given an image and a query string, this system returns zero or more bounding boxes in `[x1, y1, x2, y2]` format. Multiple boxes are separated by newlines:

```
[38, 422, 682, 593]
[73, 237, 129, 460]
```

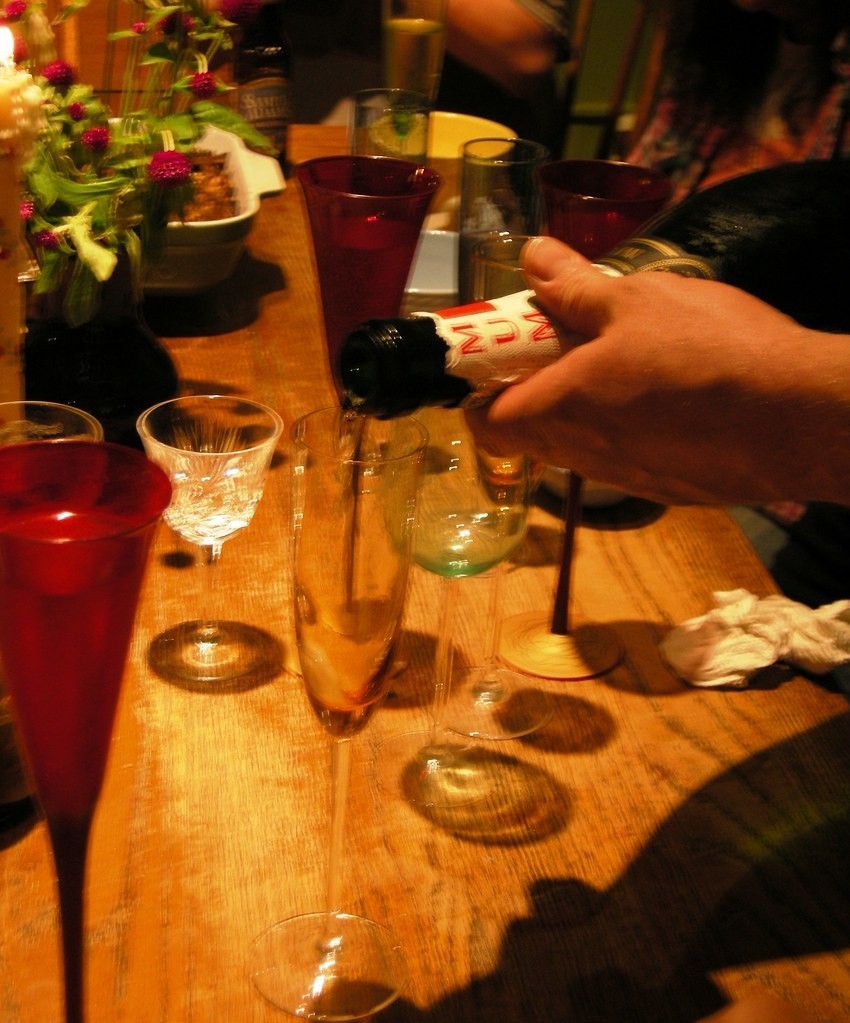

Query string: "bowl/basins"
[370, 111, 517, 159]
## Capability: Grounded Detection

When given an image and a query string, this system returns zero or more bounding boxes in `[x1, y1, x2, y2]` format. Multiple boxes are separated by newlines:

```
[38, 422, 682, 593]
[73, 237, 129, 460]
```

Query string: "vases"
[22, 252, 180, 452]
[105, 114, 290, 298]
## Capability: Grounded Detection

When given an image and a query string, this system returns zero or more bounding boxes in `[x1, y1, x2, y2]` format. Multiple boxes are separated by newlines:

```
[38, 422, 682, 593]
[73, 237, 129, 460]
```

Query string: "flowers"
[0, 0, 281, 334]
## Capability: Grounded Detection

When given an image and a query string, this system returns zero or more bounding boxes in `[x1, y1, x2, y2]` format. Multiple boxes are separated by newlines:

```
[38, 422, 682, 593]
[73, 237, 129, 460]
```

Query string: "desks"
[0, 125, 850, 1023]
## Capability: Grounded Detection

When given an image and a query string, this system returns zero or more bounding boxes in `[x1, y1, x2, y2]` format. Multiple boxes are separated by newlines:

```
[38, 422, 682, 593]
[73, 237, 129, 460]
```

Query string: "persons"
[232, 1, 579, 214]
[463, 235, 850, 694]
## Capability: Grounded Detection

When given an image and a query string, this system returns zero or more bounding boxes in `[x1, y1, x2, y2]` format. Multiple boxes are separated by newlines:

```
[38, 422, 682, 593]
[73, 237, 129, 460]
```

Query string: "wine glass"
[423, 445, 554, 741]
[137, 394, 283, 685]
[1, 442, 173, 1022]
[247, 407, 430, 1018]
[387, 414, 531, 808]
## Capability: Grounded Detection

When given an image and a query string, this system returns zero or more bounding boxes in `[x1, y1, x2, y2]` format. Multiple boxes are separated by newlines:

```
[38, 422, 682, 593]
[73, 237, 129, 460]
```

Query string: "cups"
[540, 159, 675, 258]
[297, 153, 441, 410]
[349, 87, 430, 163]
[381, 0, 448, 102]
[460, 137, 540, 303]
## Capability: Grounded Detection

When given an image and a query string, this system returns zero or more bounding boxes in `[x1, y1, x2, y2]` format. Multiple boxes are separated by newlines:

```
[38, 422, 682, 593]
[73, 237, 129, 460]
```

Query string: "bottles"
[335, 156, 849, 410]
[233, 25, 293, 154]
